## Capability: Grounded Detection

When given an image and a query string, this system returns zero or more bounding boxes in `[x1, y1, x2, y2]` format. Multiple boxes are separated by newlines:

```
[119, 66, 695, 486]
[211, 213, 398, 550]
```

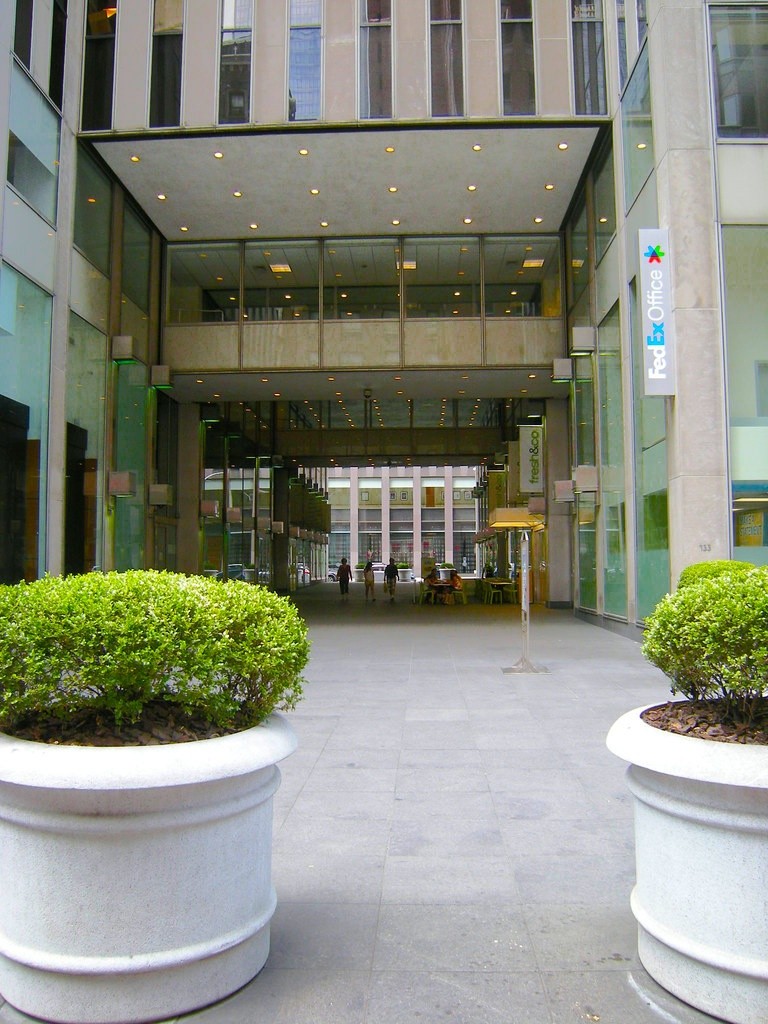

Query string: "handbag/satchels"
[383, 581, 387, 594]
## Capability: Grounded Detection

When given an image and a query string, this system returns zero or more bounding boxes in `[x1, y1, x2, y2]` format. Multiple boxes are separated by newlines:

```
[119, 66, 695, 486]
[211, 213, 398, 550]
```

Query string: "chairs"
[486, 582, 502, 605]
[418, 582, 435, 604]
[451, 581, 468, 605]
[474, 578, 482, 598]
[481, 581, 498, 603]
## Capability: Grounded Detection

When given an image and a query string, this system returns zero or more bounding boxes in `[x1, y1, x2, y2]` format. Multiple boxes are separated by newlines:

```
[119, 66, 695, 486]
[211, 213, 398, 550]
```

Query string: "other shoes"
[366, 600, 368, 602]
[443, 602, 448, 605]
[390, 597, 395, 602]
[373, 599, 376, 602]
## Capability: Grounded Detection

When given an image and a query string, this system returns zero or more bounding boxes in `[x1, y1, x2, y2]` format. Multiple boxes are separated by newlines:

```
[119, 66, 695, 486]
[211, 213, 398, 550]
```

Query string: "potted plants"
[439, 563, 456, 581]
[397, 563, 411, 582]
[0, 569, 310, 1024]
[353, 563, 365, 582]
[607, 564, 768, 1024]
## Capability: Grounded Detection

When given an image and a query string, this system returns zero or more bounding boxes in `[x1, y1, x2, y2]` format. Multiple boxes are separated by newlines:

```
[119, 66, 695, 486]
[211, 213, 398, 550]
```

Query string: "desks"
[433, 579, 450, 604]
[480, 578, 513, 603]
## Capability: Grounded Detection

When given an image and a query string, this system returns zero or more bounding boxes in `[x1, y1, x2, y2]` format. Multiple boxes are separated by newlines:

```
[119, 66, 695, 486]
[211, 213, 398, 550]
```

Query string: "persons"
[364, 562, 376, 602]
[424, 568, 437, 606]
[482, 564, 494, 579]
[337, 558, 352, 601]
[383, 558, 399, 601]
[441, 569, 462, 605]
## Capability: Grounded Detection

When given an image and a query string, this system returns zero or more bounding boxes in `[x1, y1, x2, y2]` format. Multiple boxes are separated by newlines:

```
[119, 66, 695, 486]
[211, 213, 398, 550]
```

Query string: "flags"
[489, 472, 507, 514]
[520, 427, 541, 492]
[508, 440, 530, 502]
